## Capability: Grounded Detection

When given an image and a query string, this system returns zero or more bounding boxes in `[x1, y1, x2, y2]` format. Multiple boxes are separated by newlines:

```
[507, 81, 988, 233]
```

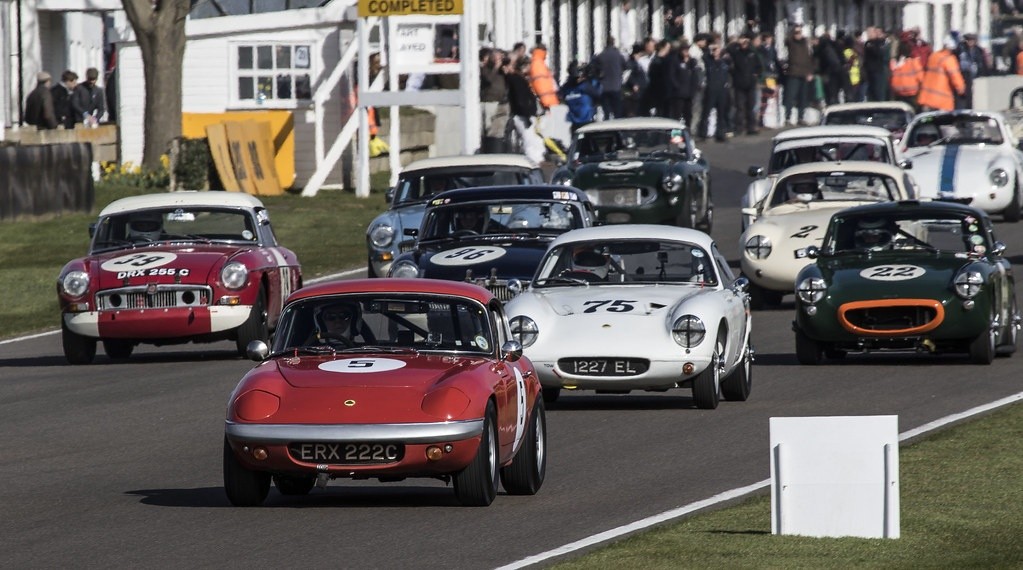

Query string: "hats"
[943, 38, 958, 50]
[964, 34, 977, 40]
[87, 68, 98, 80]
[37, 71, 52, 82]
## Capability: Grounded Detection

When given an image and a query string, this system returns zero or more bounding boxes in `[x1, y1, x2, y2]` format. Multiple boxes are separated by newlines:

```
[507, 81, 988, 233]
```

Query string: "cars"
[222, 276, 548, 509]
[386, 184, 600, 307]
[736, 158, 929, 303]
[504, 224, 754, 411]
[549, 116, 714, 232]
[893, 108, 1023, 223]
[819, 100, 917, 141]
[365, 152, 548, 280]
[790, 198, 1022, 368]
[55, 189, 304, 364]
[741, 121, 915, 234]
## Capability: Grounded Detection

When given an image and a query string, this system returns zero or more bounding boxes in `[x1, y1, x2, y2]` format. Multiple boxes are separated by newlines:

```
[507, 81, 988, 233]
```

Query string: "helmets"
[128, 212, 164, 245]
[423, 174, 454, 193]
[854, 216, 892, 251]
[454, 205, 491, 233]
[913, 123, 939, 146]
[791, 147, 822, 163]
[588, 131, 621, 153]
[570, 241, 610, 279]
[787, 174, 819, 202]
[313, 296, 363, 344]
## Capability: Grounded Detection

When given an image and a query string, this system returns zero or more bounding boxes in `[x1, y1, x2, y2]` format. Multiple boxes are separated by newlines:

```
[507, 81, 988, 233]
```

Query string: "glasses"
[324, 311, 352, 321]
[90, 78, 95, 81]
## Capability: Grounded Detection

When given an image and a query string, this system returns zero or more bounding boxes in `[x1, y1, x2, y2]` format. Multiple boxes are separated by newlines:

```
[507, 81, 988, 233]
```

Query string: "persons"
[127, 211, 165, 241]
[24, 71, 56, 133]
[787, 174, 819, 201]
[481, 0, 1023, 142]
[794, 147, 816, 163]
[855, 215, 896, 251]
[571, 246, 610, 282]
[913, 124, 940, 146]
[347, 75, 379, 193]
[312, 300, 355, 343]
[425, 177, 448, 198]
[48, 70, 78, 129]
[71, 67, 104, 125]
[454, 207, 490, 237]
